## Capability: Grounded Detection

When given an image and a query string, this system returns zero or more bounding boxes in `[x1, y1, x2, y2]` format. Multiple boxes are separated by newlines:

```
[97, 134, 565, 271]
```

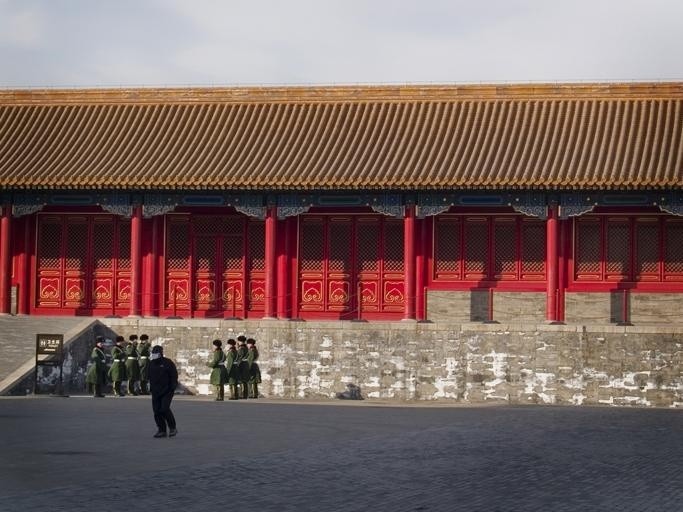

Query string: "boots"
[215, 383, 258, 401]
[94, 379, 150, 397]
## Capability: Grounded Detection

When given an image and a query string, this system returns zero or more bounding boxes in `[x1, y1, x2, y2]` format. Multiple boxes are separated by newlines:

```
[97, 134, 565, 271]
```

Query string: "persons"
[126, 335, 141, 396]
[107, 335, 129, 397]
[236, 336, 250, 399]
[87, 336, 109, 397]
[225, 339, 239, 400]
[246, 338, 262, 399]
[147, 345, 179, 438]
[205, 339, 227, 401]
[136, 334, 152, 395]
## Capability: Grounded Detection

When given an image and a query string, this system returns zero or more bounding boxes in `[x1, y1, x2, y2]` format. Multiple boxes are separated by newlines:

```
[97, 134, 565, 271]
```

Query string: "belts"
[128, 357, 138, 360]
[233, 362, 238, 365]
[242, 358, 248, 362]
[101, 360, 106, 364]
[218, 362, 225, 365]
[114, 358, 125, 362]
[141, 356, 148, 359]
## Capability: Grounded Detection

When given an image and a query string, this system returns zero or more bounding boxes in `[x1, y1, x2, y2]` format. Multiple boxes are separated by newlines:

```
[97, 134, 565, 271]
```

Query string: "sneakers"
[169, 429, 176, 436]
[153, 431, 166, 437]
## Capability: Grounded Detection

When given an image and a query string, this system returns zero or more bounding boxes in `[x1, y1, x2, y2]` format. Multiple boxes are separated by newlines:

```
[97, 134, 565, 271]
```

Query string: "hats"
[247, 339, 256, 344]
[96, 335, 105, 343]
[238, 336, 247, 342]
[228, 339, 235, 345]
[214, 340, 222, 346]
[130, 335, 137, 340]
[152, 346, 163, 353]
[140, 335, 148, 340]
[116, 336, 123, 343]
